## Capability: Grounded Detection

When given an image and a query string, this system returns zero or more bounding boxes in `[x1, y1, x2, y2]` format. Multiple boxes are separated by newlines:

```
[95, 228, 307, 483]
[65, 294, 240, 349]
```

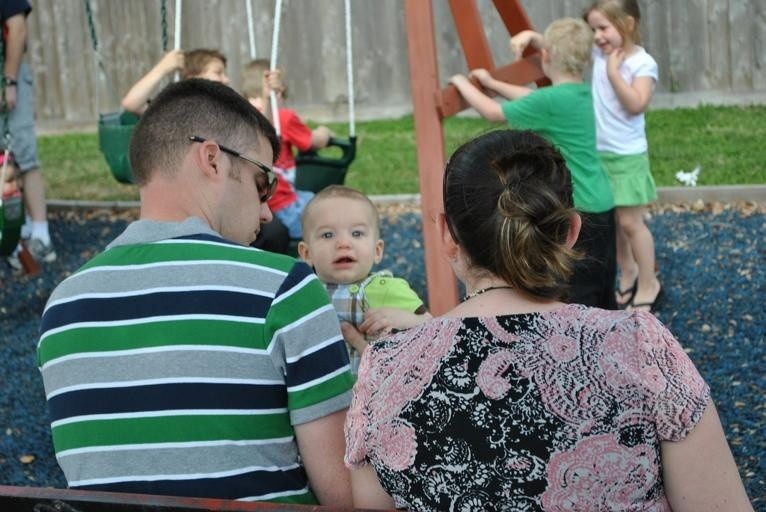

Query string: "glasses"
[189, 135, 277, 201]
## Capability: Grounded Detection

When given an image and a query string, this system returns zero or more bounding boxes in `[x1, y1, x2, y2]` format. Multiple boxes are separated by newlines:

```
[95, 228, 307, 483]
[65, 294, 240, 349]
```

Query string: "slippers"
[616, 278, 637, 303]
[631, 287, 663, 312]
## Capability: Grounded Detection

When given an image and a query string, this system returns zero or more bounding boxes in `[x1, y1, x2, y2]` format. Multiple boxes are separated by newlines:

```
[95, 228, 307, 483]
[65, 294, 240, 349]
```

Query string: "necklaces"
[459, 285, 517, 303]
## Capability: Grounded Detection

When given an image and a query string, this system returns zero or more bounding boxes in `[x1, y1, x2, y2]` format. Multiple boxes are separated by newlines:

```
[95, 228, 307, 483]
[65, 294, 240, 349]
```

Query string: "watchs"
[3, 77, 17, 85]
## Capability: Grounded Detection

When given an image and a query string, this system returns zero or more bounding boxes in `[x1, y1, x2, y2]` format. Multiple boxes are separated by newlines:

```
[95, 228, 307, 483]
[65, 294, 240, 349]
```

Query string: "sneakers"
[6, 239, 56, 269]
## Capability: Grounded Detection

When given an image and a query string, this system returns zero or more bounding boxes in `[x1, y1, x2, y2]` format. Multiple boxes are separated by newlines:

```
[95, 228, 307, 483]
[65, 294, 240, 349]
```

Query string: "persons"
[510, 0, 665, 316]
[239, 59, 346, 237]
[120, 48, 291, 258]
[341, 129, 757, 512]
[0, 0, 57, 266]
[297, 182, 432, 375]
[447, 17, 616, 313]
[33, 76, 369, 510]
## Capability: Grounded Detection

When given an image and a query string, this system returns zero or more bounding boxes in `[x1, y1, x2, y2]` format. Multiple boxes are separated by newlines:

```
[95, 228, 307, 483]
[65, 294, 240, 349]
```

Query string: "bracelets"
[154, 63, 170, 75]
